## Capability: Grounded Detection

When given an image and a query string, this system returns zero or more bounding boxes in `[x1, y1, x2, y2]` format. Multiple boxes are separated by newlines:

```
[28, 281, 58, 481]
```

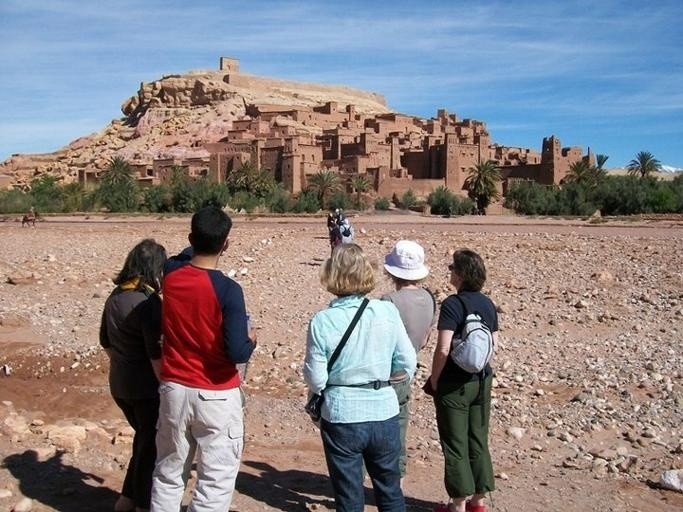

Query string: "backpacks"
[342, 226, 351, 237]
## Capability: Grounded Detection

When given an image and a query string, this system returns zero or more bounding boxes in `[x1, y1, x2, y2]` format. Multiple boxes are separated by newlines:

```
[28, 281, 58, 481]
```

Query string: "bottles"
[236, 314, 253, 381]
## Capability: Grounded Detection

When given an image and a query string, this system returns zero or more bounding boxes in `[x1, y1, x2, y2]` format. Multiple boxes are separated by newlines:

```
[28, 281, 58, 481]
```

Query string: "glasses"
[449, 263, 456, 271]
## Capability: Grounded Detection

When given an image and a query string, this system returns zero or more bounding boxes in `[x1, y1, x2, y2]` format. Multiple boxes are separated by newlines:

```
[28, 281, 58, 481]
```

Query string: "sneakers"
[434, 502, 453, 512]
[466, 499, 486, 512]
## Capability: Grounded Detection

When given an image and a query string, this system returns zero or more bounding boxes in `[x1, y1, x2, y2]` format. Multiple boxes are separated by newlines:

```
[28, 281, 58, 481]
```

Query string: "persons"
[99, 238, 166, 512]
[361, 239, 436, 489]
[303, 241, 419, 512]
[421, 247, 499, 512]
[144, 204, 258, 511]
[28, 206, 34, 217]
[326, 207, 355, 250]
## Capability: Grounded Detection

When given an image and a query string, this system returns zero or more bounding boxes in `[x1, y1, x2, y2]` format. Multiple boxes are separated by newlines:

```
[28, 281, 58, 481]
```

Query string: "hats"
[384, 241, 429, 280]
[341, 219, 348, 224]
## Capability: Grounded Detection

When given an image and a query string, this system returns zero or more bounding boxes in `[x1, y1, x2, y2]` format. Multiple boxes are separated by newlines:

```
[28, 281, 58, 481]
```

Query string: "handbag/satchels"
[449, 294, 493, 375]
[305, 387, 324, 429]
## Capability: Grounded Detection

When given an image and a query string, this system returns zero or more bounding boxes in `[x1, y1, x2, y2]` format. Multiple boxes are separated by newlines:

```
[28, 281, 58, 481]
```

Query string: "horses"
[22, 211, 42, 227]
